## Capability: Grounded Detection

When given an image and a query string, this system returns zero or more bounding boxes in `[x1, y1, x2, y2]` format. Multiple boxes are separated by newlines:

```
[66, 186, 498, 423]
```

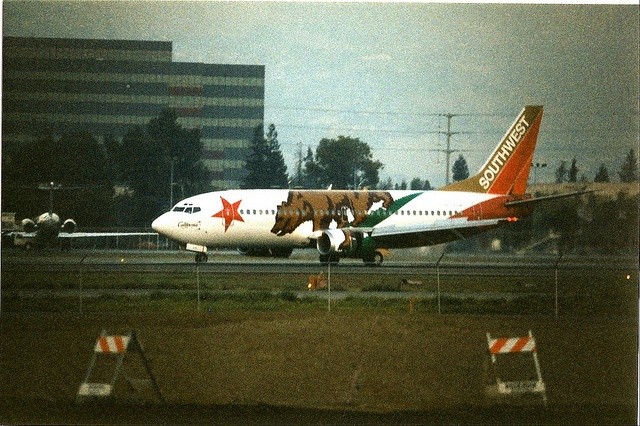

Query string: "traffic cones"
[72, 328, 165, 405]
[483, 330, 547, 405]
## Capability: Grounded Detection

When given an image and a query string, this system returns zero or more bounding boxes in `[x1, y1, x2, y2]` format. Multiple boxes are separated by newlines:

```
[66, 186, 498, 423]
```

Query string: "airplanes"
[151, 105, 604, 266]
[21, 210, 80, 248]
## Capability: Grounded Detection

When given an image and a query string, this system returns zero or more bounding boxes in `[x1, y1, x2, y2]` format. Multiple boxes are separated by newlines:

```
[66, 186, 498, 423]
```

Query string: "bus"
[4, 231, 160, 253]
